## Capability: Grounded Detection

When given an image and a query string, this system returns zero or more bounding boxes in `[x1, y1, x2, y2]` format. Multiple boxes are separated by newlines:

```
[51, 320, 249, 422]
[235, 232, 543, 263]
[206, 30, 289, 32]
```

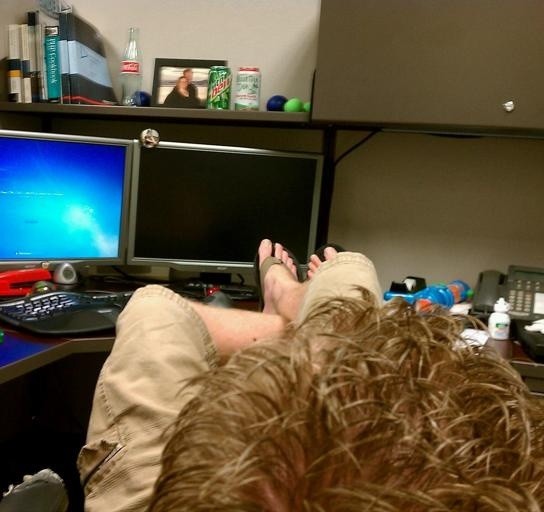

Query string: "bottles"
[118, 25, 142, 107]
[448, 280, 471, 304]
[413, 284, 454, 316]
[489, 296, 511, 341]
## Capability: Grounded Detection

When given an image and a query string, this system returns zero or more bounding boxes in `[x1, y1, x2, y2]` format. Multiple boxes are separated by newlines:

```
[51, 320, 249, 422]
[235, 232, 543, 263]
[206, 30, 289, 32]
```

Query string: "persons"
[182, 69, 199, 105]
[163, 77, 201, 107]
[73, 236, 543, 512]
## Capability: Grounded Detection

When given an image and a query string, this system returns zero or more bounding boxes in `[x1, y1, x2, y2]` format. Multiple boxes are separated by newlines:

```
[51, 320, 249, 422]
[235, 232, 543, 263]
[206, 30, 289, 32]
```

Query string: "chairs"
[0, 479, 69, 512]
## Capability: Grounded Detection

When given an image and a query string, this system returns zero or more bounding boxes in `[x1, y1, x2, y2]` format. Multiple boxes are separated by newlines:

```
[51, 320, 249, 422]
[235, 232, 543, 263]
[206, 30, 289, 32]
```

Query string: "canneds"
[206, 65, 232, 109]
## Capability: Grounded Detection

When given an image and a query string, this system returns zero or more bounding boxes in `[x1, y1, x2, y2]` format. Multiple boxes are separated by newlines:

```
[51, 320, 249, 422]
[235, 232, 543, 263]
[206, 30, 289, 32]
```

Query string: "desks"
[1, 298, 544, 405]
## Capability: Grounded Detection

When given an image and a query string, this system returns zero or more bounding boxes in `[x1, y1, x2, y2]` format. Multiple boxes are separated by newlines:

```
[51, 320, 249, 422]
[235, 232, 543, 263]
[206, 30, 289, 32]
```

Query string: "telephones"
[469, 266, 544, 322]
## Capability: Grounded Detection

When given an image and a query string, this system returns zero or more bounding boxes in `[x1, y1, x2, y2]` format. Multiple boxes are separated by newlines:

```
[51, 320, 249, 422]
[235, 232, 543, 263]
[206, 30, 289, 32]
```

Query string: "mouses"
[202, 290, 233, 308]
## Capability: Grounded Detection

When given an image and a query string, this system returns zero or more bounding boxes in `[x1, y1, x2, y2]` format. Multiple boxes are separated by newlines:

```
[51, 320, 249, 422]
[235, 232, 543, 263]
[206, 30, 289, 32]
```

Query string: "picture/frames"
[149, 58, 226, 109]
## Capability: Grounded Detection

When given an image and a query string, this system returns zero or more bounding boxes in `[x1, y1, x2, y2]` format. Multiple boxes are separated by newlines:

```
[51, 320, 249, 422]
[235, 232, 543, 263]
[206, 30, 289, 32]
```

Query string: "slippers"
[254, 242, 304, 313]
[306, 244, 344, 283]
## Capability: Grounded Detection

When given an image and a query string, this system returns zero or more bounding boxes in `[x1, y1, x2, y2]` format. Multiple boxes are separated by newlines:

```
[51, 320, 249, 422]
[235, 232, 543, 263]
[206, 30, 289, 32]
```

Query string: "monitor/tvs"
[0, 130, 133, 290]
[126, 138, 325, 301]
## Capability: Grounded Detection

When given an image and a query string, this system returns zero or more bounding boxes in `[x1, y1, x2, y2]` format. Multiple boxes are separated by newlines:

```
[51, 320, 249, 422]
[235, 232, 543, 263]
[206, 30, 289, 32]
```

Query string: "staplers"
[1, 269, 53, 296]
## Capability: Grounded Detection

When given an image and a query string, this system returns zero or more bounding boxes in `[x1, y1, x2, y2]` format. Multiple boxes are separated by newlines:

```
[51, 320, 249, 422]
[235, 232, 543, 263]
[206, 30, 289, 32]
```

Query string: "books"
[6, 9, 120, 107]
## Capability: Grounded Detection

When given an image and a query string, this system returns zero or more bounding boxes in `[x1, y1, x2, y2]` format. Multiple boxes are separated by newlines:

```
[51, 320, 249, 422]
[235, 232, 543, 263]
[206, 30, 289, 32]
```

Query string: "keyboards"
[0, 289, 201, 336]
